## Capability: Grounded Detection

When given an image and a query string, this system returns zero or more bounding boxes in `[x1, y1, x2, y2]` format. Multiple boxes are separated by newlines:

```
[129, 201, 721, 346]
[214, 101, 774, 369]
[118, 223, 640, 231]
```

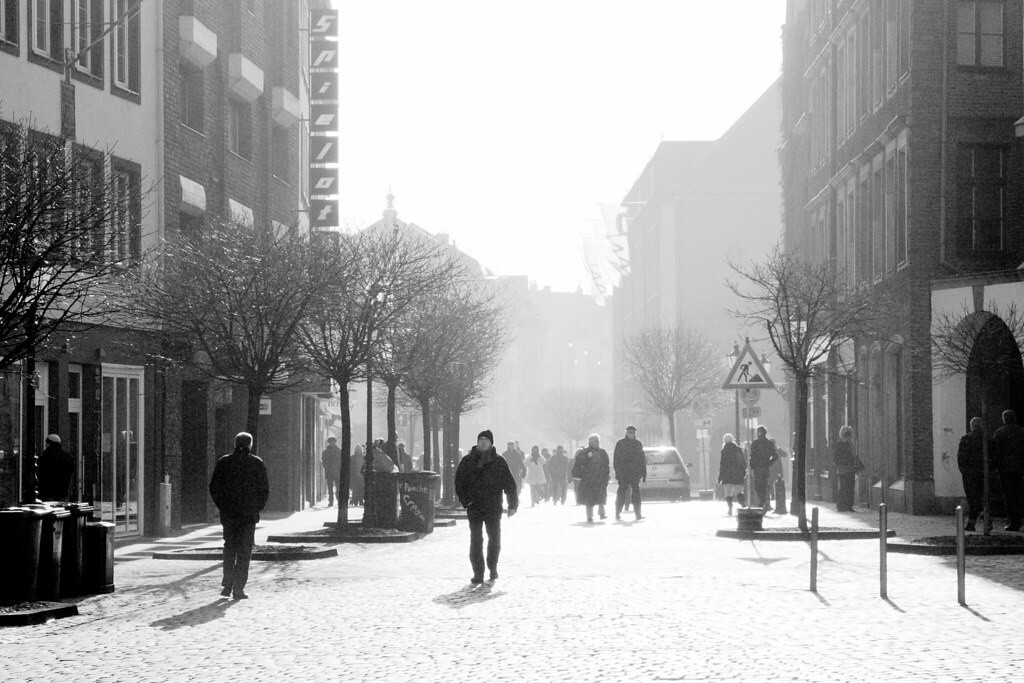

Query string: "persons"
[502, 441, 525, 499]
[613, 426, 647, 520]
[717, 434, 747, 514]
[524, 445, 546, 506]
[36, 434, 74, 501]
[321, 437, 341, 507]
[350, 432, 412, 505]
[833, 426, 855, 512]
[993, 410, 1024, 532]
[541, 448, 552, 502]
[572, 434, 610, 521]
[568, 446, 586, 506]
[455, 430, 518, 582]
[750, 427, 777, 507]
[210, 432, 269, 599]
[547, 445, 572, 504]
[957, 417, 994, 532]
[735, 439, 788, 500]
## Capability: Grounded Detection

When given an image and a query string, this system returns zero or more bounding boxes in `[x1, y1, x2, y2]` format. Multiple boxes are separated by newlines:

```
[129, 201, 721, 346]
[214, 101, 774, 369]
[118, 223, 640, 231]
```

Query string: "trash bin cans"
[22, 504, 72, 600]
[396, 471, 440, 533]
[50, 502, 94, 597]
[0, 507, 50, 604]
[360, 469, 397, 528]
[84, 521, 116, 594]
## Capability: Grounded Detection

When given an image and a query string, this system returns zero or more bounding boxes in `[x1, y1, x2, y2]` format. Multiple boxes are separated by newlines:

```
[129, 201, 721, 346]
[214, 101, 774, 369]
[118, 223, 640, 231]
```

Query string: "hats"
[626, 426, 636, 430]
[477, 429, 493, 445]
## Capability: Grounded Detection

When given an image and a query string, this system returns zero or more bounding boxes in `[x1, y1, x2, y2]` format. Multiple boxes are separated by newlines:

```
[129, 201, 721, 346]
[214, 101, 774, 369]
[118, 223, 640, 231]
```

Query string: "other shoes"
[838, 507, 855, 512]
[637, 516, 645, 520]
[221, 588, 231, 596]
[600, 515, 607, 520]
[965, 527, 976, 531]
[233, 593, 248, 599]
[587, 517, 593, 522]
[489, 564, 498, 579]
[471, 578, 484, 584]
[1004, 522, 1016, 531]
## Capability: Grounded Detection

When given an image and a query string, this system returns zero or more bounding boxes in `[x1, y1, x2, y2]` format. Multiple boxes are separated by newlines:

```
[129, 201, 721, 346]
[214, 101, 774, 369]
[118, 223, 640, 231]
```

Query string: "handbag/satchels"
[572, 449, 588, 479]
[849, 455, 865, 473]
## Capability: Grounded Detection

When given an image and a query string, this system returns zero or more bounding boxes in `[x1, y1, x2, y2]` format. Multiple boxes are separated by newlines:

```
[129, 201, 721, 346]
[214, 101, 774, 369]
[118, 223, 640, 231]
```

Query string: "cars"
[630, 445, 693, 499]
